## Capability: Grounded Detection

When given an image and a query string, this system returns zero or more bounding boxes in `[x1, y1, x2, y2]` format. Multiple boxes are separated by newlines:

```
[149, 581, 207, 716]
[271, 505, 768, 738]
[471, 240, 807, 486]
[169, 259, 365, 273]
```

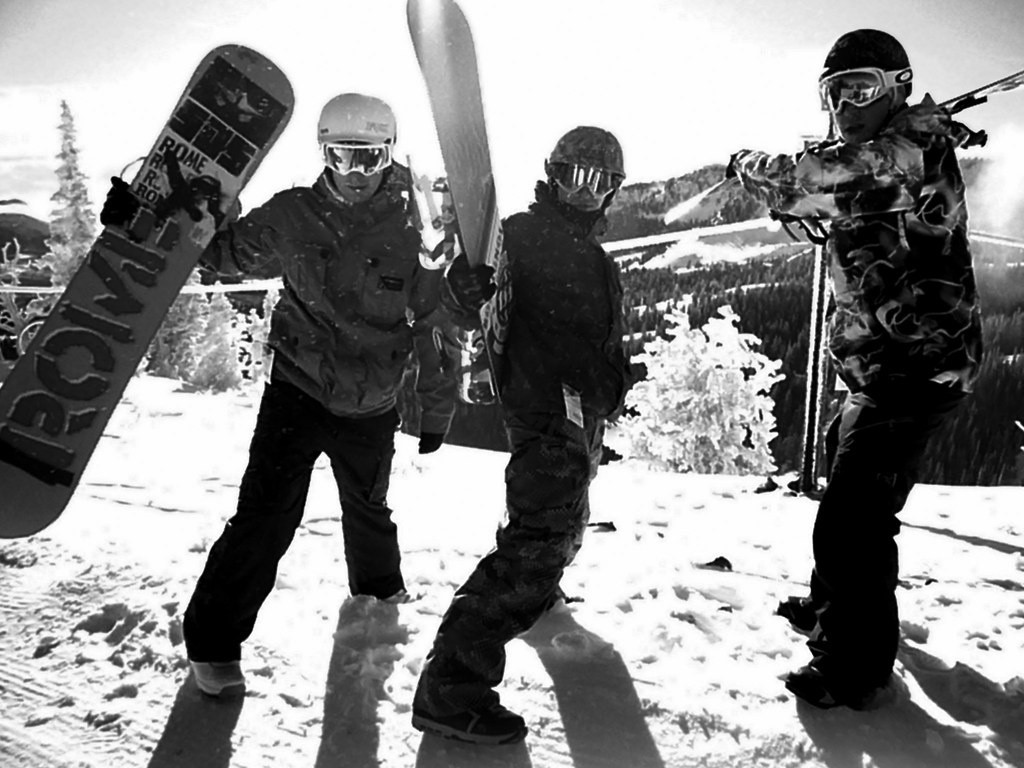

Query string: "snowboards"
[1, 44, 295, 540]
[663, 68, 1022, 226]
[406, 0, 516, 453]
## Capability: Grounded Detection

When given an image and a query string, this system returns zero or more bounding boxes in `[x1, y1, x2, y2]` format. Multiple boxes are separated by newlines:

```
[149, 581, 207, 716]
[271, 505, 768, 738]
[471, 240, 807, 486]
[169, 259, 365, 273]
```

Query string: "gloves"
[100, 176, 138, 226]
[726, 153, 741, 178]
[449, 263, 484, 300]
[418, 431, 445, 454]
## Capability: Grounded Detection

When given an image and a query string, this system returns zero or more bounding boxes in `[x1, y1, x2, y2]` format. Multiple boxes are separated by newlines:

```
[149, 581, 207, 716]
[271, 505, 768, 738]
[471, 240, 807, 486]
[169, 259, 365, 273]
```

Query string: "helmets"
[819, 29, 912, 99]
[544, 126, 625, 210]
[317, 93, 397, 150]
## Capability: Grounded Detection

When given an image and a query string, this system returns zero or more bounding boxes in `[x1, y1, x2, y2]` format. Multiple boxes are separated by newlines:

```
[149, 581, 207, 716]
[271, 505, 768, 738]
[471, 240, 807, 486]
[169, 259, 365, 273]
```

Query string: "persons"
[100, 93, 498, 701]
[725, 28, 982, 710]
[413, 127, 632, 745]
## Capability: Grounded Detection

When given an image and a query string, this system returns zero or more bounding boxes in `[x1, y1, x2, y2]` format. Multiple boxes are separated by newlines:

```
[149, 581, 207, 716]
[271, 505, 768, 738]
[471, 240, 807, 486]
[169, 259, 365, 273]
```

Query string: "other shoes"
[776, 596, 818, 635]
[784, 665, 878, 712]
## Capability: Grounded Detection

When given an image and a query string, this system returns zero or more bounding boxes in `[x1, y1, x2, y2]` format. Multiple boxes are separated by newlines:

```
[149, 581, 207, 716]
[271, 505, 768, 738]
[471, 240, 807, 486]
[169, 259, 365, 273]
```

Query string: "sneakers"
[411, 690, 528, 744]
[189, 658, 246, 706]
[546, 596, 584, 611]
[384, 588, 428, 604]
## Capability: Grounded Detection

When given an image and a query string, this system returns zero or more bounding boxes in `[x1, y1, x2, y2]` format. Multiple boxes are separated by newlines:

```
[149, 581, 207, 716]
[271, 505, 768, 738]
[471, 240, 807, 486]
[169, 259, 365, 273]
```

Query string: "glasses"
[322, 136, 393, 176]
[818, 66, 889, 113]
[543, 159, 626, 198]
